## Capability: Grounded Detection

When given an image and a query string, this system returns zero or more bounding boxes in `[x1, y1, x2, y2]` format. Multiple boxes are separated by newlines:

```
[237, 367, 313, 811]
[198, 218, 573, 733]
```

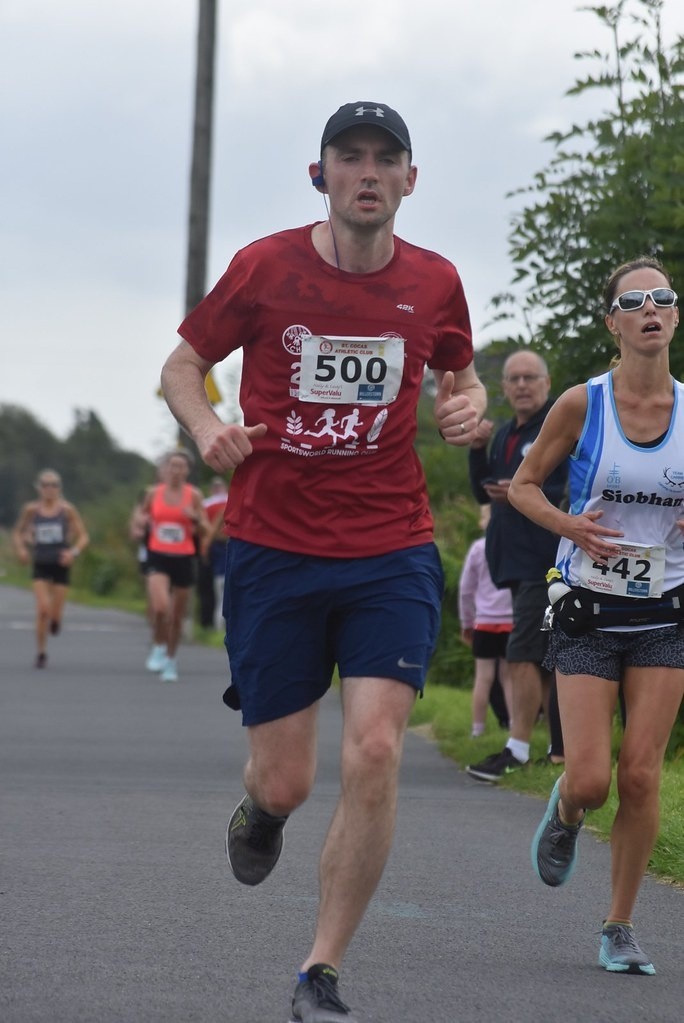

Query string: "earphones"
[312, 175, 324, 187]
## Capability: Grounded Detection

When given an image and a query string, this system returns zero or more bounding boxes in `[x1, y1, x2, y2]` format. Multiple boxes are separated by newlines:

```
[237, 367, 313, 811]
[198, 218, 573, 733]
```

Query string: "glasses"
[503, 374, 546, 383]
[609, 288, 679, 315]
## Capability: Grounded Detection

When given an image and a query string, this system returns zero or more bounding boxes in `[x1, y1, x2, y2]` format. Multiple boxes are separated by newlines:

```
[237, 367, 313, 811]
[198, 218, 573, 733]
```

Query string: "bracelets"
[70, 546, 81, 557]
[192, 513, 200, 525]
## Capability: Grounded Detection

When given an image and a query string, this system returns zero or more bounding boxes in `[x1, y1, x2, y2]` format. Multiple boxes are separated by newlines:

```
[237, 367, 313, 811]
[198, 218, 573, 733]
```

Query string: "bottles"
[138, 544, 148, 563]
[546, 570, 584, 606]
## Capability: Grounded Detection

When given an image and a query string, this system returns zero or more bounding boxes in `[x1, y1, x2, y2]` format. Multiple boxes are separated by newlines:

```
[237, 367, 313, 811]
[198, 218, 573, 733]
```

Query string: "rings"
[459, 424, 465, 435]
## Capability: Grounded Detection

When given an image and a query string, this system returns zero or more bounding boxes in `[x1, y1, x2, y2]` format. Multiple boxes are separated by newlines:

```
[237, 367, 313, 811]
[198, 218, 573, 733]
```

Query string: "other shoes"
[534, 752, 564, 766]
[33, 653, 47, 669]
[146, 644, 178, 683]
[50, 620, 58, 634]
[465, 748, 530, 782]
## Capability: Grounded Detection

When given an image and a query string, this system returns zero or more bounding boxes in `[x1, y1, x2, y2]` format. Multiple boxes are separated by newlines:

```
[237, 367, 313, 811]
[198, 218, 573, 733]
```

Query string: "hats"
[321, 101, 411, 151]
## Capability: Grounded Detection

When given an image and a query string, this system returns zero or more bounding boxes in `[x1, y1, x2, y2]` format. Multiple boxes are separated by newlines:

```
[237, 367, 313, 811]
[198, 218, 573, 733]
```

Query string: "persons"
[14, 469, 90, 670]
[455, 349, 566, 783]
[160, 105, 487, 1023]
[508, 259, 684, 975]
[131, 453, 228, 683]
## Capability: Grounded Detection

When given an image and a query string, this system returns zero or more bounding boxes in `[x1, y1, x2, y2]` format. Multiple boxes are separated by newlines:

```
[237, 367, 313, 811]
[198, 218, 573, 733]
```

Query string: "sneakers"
[225, 794, 290, 885]
[594, 920, 656, 975]
[531, 777, 586, 887]
[288, 964, 354, 1023]
